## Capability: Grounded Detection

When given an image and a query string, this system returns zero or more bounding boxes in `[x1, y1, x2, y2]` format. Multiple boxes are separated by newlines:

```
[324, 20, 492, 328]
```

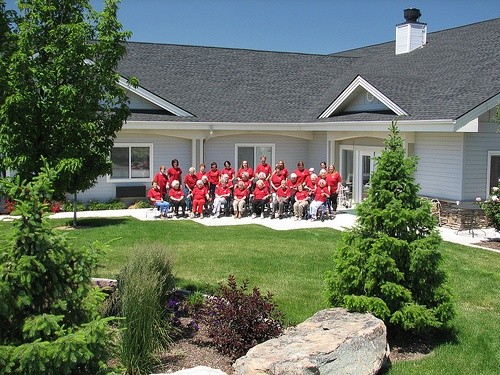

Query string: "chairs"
[166, 191, 331, 221]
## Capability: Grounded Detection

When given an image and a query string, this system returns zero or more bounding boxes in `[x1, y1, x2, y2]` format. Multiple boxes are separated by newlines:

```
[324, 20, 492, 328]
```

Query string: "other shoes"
[212, 207, 217, 213]
[307, 215, 317, 221]
[294, 217, 302, 221]
[271, 214, 275, 219]
[260, 213, 264, 219]
[333, 211, 336, 215]
[252, 212, 257, 218]
[191, 212, 194, 218]
[217, 211, 221, 217]
[279, 215, 282, 219]
[160, 212, 172, 219]
[234, 211, 242, 219]
[176, 213, 186, 218]
[200, 213, 203, 219]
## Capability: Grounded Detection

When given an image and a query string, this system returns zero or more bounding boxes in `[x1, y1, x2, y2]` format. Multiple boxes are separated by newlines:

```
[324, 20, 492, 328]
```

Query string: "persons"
[147, 157, 340, 222]
